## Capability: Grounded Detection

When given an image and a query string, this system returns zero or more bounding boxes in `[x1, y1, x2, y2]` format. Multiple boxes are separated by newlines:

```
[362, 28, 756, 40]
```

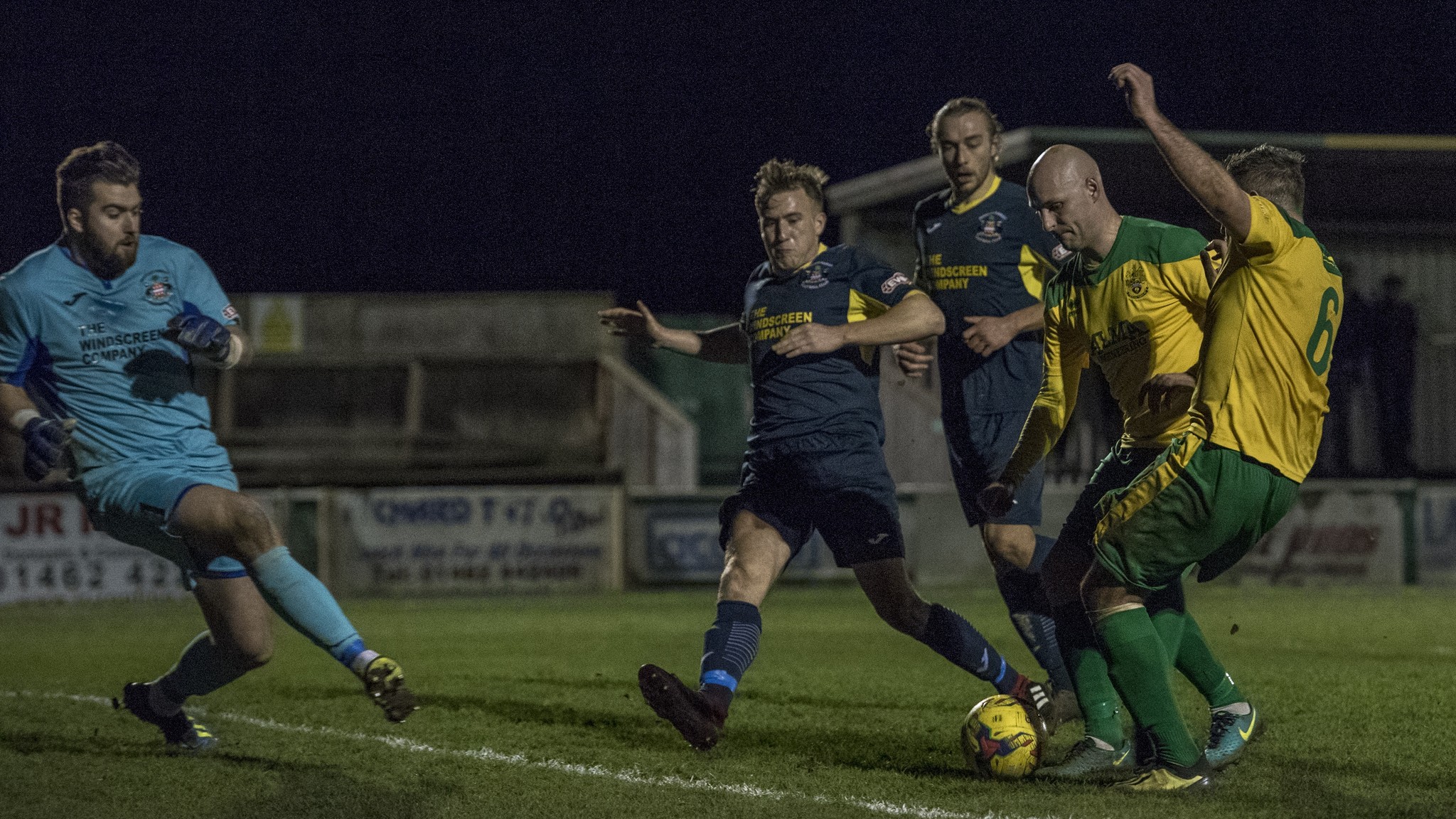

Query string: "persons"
[597, 159, 1056, 749]
[890, 97, 1083, 734]
[0, 141, 417, 748]
[972, 142, 1266, 785]
[1079, 63, 1343, 791]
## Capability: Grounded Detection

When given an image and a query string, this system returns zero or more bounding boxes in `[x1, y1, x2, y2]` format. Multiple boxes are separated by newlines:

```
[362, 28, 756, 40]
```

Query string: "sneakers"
[1202, 697, 1265, 771]
[1050, 685, 1080, 721]
[1018, 678, 1056, 736]
[123, 681, 219, 749]
[1113, 761, 1216, 793]
[363, 654, 414, 722]
[636, 662, 726, 750]
[1034, 737, 1136, 783]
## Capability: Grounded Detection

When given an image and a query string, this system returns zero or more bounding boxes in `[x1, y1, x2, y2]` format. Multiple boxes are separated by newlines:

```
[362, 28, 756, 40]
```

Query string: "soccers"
[961, 695, 1049, 780]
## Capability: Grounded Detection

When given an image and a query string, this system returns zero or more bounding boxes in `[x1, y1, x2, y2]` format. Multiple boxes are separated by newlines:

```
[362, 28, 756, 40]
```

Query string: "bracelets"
[9, 408, 40, 433]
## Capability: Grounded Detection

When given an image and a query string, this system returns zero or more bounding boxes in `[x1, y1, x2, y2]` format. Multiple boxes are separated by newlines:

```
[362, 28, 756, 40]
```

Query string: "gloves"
[24, 417, 82, 483]
[162, 313, 232, 361]
